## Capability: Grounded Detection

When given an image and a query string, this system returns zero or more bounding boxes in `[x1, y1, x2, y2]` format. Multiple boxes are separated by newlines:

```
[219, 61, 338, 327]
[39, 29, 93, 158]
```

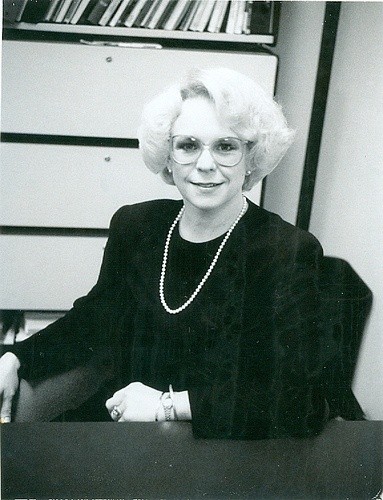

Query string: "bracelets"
[155, 392, 179, 423]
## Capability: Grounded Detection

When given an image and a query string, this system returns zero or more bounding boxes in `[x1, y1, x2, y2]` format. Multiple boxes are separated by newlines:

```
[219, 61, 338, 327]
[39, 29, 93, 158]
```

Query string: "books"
[3, 0, 273, 37]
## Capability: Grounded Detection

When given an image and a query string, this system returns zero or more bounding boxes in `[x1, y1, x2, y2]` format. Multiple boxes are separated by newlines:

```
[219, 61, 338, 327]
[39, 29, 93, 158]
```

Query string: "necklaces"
[159, 193, 248, 315]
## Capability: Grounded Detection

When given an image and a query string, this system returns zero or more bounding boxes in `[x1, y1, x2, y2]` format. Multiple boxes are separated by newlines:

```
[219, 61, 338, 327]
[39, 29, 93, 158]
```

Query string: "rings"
[113, 406, 122, 417]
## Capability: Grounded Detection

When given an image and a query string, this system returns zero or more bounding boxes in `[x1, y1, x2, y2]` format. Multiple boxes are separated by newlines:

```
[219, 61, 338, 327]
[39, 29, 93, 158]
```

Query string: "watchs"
[163, 396, 173, 421]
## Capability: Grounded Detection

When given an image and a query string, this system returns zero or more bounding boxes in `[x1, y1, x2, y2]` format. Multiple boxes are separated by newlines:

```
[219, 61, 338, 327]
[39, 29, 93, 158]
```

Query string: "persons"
[1, 68, 334, 439]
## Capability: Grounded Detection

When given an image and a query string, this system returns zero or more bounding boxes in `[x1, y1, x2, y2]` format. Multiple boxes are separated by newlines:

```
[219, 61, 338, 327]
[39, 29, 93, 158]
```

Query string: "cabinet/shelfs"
[2, 0, 277, 349]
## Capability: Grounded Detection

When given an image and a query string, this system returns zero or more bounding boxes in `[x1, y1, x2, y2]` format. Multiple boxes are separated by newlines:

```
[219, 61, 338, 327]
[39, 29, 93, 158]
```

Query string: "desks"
[0, 421, 383, 500]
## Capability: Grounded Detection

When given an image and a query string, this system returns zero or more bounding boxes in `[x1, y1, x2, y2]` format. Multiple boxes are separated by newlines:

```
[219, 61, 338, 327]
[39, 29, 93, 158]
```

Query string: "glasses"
[169, 134, 253, 166]
